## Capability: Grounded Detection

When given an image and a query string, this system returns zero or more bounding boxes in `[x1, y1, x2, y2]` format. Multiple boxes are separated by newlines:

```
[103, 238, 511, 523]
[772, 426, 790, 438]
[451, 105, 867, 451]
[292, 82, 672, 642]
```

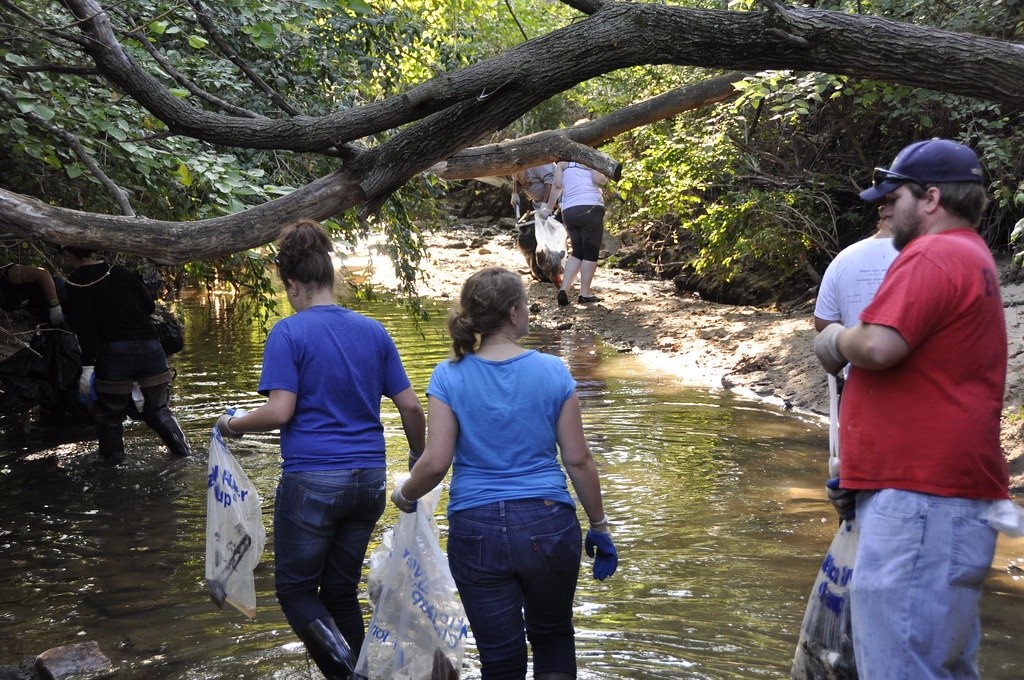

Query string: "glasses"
[870, 165, 929, 191]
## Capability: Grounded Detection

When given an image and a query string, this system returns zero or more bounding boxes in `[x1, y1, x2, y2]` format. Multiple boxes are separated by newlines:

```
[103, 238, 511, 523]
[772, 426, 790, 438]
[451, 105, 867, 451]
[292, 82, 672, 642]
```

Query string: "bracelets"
[51, 303, 58, 308]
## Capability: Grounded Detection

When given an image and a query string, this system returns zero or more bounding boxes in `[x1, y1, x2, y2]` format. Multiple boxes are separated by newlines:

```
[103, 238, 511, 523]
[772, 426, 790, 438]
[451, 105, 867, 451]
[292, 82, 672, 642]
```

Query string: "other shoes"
[578, 294, 600, 303]
[556, 290, 569, 306]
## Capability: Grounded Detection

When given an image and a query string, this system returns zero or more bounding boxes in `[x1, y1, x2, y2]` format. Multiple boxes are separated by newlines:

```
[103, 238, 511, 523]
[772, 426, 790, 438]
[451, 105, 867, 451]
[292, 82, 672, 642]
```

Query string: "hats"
[857, 135, 984, 203]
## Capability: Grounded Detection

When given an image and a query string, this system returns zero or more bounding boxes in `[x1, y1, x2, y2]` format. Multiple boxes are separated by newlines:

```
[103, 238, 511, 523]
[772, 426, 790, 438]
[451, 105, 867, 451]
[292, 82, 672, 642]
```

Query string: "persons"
[814, 138, 1012, 680]
[215, 221, 427, 680]
[391, 267, 618, 680]
[0, 261, 71, 343]
[512, 162, 563, 224]
[539, 119, 607, 305]
[61, 246, 190, 455]
[814, 199, 900, 379]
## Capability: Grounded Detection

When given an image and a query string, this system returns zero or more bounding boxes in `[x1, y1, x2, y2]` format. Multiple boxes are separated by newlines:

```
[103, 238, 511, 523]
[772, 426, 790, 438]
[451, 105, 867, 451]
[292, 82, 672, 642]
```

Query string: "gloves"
[390, 483, 417, 514]
[511, 193, 521, 208]
[824, 476, 854, 521]
[539, 208, 552, 220]
[50, 303, 65, 325]
[407, 449, 420, 471]
[812, 322, 847, 379]
[215, 414, 245, 440]
[584, 517, 618, 581]
[78, 366, 97, 404]
